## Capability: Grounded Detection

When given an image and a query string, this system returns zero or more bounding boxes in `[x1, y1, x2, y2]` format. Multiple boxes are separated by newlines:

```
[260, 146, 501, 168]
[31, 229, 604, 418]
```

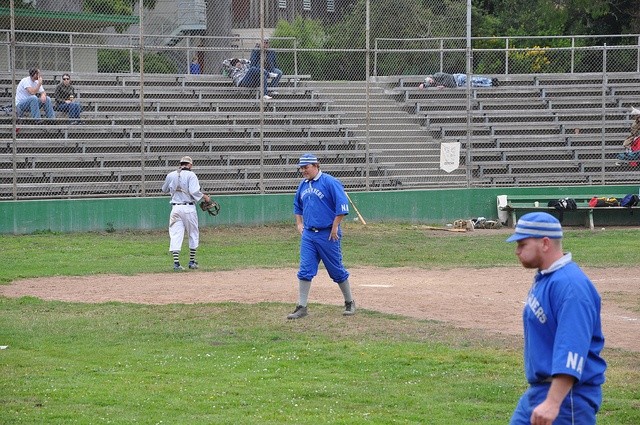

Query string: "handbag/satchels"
[589, 195, 608, 207]
[548, 198, 577, 211]
[620, 194, 640, 207]
[603, 196, 618, 206]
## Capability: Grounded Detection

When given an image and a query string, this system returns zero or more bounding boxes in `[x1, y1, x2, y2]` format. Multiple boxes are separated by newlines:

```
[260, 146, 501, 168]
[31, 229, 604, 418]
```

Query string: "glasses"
[63, 78, 69, 80]
[635, 120, 640, 125]
[265, 42, 271, 45]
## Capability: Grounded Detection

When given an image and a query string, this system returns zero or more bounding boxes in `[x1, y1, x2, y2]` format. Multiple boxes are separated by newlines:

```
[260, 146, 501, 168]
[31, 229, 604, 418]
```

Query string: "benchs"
[371, 73, 639, 185]
[498, 195, 640, 229]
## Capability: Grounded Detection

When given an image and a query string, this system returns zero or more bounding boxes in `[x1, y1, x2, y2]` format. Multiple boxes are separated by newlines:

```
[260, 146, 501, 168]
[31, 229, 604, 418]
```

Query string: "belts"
[539, 377, 552, 384]
[173, 202, 194, 205]
[310, 225, 331, 231]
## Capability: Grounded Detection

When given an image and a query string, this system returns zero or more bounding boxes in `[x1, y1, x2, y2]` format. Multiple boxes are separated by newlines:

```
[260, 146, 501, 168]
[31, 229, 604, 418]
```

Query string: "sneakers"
[189, 261, 199, 269]
[173, 263, 185, 271]
[81, 121, 86, 125]
[287, 304, 308, 320]
[69, 121, 78, 125]
[493, 82, 500, 86]
[264, 95, 272, 99]
[343, 300, 356, 316]
[268, 90, 280, 95]
[492, 78, 498, 81]
[268, 73, 278, 78]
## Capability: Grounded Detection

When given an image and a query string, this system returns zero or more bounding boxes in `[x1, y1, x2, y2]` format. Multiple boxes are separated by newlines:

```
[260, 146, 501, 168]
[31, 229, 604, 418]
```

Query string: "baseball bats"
[418, 224, 467, 233]
[345, 192, 366, 225]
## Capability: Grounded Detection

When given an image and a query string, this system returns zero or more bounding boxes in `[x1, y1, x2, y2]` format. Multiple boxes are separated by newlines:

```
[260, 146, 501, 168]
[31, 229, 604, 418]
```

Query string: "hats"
[180, 156, 193, 165]
[231, 59, 240, 66]
[298, 154, 319, 167]
[505, 212, 563, 242]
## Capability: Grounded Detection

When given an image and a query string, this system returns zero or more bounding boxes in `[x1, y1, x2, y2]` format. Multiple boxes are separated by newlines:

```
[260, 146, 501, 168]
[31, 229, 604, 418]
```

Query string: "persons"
[189, 57, 200, 74]
[264, 38, 283, 85]
[419, 72, 500, 88]
[222, 57, 272, 100]
[617, 116, 640, 169]
[15, 68, 57, 118]
[286, 153, 357, 319]
[250, 43, 261, 67]
[53, 73, 86, 126]
[507, 211, 608, 425]
[161, 155, 211, 270]
